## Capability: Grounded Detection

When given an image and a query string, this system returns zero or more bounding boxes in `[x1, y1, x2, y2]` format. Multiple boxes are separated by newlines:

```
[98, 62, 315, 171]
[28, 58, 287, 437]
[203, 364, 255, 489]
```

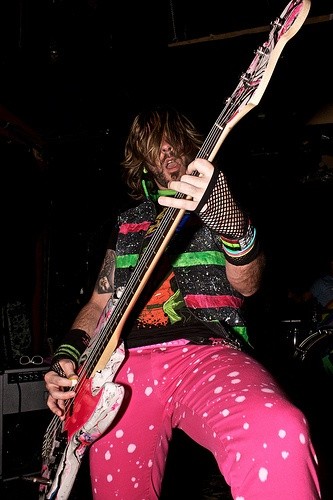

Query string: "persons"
[44, 108, 323, 500]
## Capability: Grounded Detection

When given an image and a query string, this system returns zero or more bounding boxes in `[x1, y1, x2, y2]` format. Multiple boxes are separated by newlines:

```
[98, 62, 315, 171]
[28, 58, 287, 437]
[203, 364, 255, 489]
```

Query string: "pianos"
[0, 361, 55, 419]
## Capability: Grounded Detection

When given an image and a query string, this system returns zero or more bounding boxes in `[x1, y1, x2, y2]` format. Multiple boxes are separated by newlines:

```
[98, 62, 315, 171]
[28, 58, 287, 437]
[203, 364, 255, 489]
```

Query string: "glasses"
[18, 355, 45, 365]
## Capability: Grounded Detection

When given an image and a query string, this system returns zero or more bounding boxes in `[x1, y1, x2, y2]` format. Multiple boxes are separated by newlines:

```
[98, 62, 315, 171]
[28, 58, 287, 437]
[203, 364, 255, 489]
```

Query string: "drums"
[292, 328, 332, 364]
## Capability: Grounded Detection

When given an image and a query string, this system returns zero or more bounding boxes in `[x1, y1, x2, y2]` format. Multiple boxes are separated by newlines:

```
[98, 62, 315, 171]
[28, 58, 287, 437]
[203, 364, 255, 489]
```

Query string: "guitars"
[36, 0, 313, 500]
